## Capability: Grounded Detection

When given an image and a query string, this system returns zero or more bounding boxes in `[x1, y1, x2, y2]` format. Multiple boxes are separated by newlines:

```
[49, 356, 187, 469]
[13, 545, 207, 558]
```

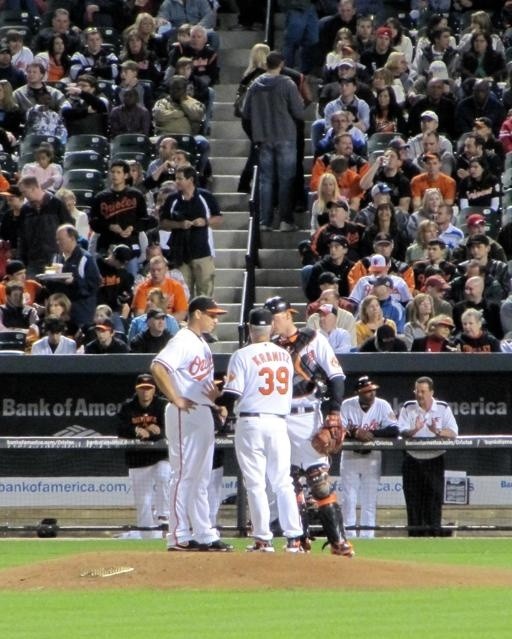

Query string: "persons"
[149, 296, 233, 553]
[207, 398, 231, 528]
[201, 306, 311, 553]
[262, 296, 354, 557]
[0, 1, 512, 352]
[338, 375, 399, 536]
[112, 374, 171, 540]
[397, 376, 459, 537]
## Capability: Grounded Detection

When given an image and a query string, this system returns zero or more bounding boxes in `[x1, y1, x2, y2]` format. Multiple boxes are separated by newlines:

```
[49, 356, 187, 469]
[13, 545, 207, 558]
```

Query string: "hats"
[134, 371, 157, 391]
[186, 295, 229, 316]
[93, 317, 115, 332]
[417, 109, 493, 166]
[315, 303, 343, 319]
[145, 307, 169, 321]
[317, 228, 458, 293]
[323, 198, 349, 212]
[264, 296, 302, 316]
[246, 305, 274, 326]
[354, 373, 379, 394]
[429, 314, 455, 330]
[466, 212, 490, 227]
[368, 180, 398, 195]
[329, 25, 450, 85]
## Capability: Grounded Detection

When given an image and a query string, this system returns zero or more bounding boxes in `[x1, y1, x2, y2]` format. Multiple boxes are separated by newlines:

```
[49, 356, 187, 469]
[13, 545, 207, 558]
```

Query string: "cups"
[53, 263, 64, 273]
[45, 266, 57, 274]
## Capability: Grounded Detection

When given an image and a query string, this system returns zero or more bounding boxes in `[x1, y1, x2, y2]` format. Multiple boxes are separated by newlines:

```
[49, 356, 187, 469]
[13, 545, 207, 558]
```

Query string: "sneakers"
[258, 222, 275, 233]
[284, 537, 313, 554]
[197, 540, 234, 553]
[156, 514, 171, 527]
[280, 220, 301, 233]
[321, 537, 355, 557]
[243, 540, 274, 554]
[168, 539, 197, 552]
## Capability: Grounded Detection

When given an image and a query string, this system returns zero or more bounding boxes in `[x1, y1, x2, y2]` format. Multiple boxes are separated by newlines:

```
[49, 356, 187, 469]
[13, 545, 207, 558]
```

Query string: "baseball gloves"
[311, 415, 349, 456]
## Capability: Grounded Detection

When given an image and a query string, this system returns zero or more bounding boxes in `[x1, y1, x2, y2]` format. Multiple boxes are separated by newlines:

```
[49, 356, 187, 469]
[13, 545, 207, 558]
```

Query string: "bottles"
[167, 166, 176, 174]
[382, 159, 389, 165]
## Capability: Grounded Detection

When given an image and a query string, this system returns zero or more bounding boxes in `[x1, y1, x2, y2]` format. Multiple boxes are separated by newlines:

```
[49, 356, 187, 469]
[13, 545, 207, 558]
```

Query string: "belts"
[240, 411, 285, 419]
[289, 406, 313, 413]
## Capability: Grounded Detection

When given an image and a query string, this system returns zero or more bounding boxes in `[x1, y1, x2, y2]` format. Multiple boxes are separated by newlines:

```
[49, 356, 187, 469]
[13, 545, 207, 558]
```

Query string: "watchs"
[437, 428, 442, 436]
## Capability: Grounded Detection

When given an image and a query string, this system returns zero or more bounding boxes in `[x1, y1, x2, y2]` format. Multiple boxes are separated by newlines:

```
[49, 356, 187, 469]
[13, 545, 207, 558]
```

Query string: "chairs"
[307, 3, 509, 351]
[1, 0, 221, 353]
